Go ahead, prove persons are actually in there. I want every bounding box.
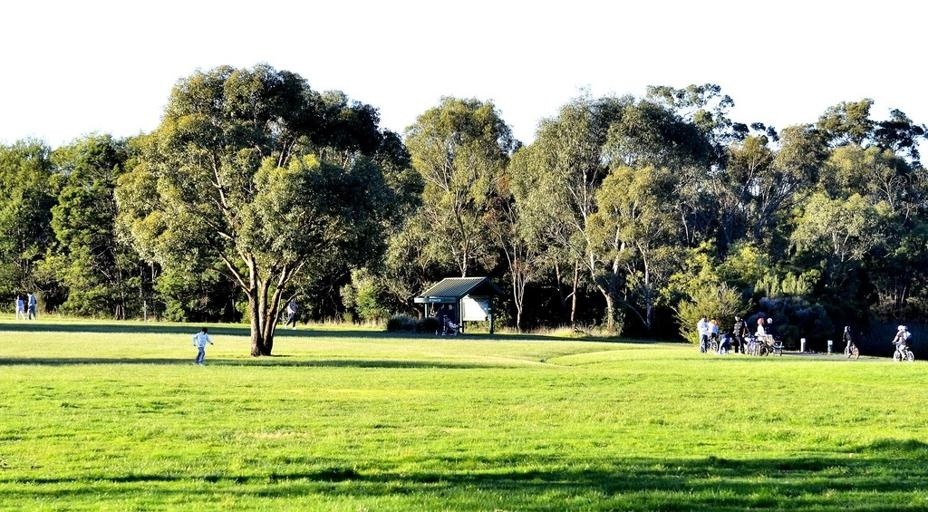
[843,325,856,358]
[892,325,911,360]
[192,327,214,368]
[26,291,37,320]
[284,297,298,330]
[15,293,28,321]
[695,313,784,355]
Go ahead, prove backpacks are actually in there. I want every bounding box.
[903,331,912,341]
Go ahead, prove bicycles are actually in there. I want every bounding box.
[891,341,914,362]
[706,331,784,360]
[841,340,860,361]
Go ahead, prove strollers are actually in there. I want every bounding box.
[442,314,463,338]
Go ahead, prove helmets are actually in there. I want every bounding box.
[897,325,908,330]
